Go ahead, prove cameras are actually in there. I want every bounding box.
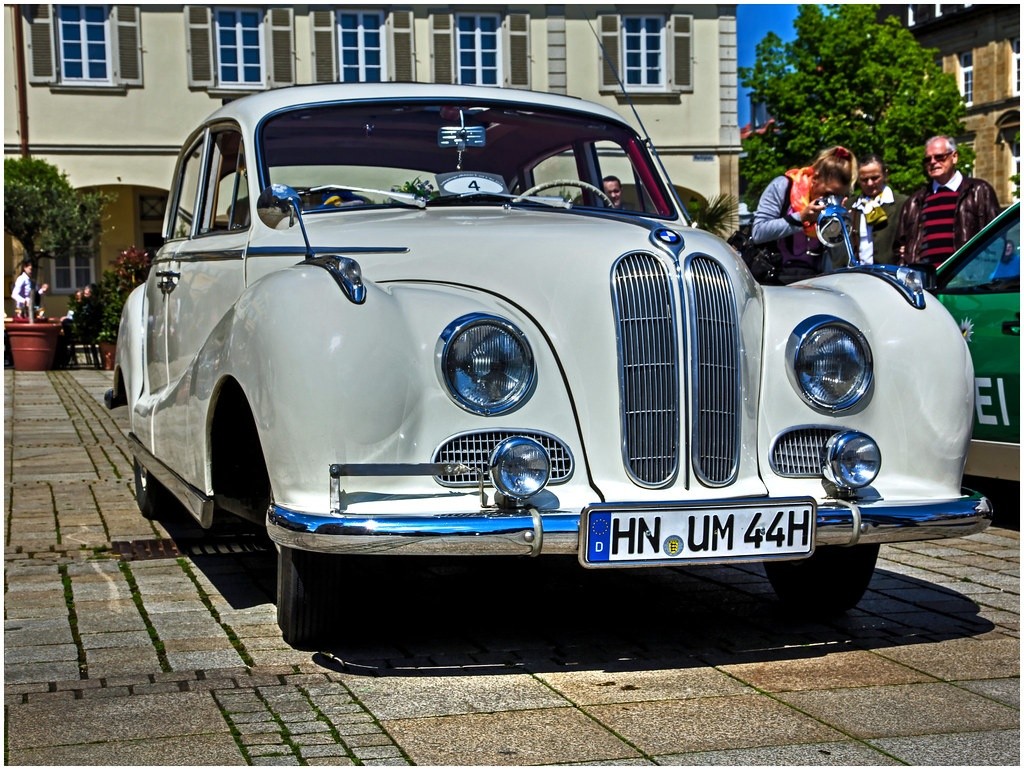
[817,194,842,209]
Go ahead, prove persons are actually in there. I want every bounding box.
[752,136,1020,287]
[603,175,634,210]
[10,260,109,369]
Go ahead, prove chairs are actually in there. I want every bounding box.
[67,342,101,370]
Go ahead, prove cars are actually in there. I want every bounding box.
[930,198,1020,480]
[102,79,994,652]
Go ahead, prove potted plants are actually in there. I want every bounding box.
[4,156,118,371]
[98,268,132,370]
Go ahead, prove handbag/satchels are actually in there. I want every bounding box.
[727,175,793,285]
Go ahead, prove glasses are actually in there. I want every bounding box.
[923,151,952,165]
